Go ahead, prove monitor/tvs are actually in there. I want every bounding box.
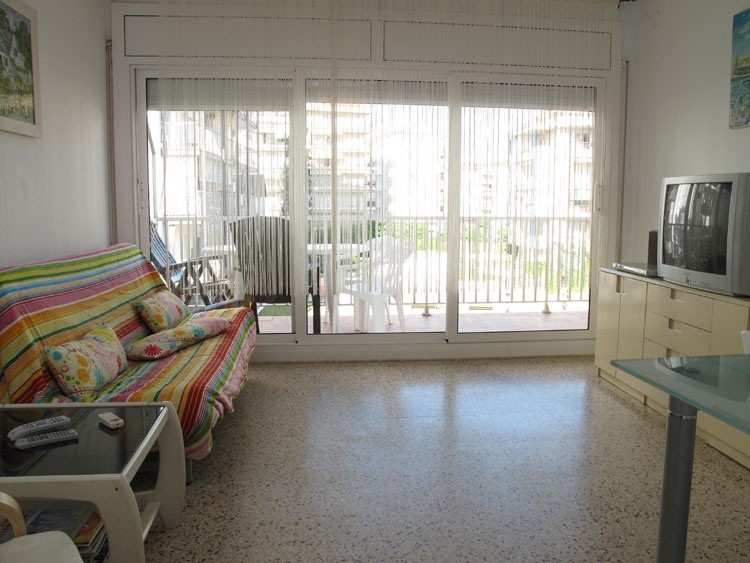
[658,171,750,297]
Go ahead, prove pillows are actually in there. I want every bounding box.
[125,316,231,360]
[43,322,128,403]
[132,290,193,333]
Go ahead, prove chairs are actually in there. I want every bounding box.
[327,236,417,331]
[149,220,234,306]
[229,217,322,335]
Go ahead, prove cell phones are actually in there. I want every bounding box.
[97,412,125,430]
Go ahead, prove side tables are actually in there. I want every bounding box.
[0,403,188,563]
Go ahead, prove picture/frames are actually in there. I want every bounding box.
[0,0,43,139]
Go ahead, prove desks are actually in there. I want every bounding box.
[202,243,370,327]
[610,355,750,563]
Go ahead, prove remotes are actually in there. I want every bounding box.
[14,429,78,449]
[6,415,71,442]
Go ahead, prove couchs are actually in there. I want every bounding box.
[0,243,257,484]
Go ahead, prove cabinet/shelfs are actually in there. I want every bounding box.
[594,271,647,406]
[644,283,715,439]
[713,299,750,469]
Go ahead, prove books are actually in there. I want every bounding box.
[0,509,109,563]
